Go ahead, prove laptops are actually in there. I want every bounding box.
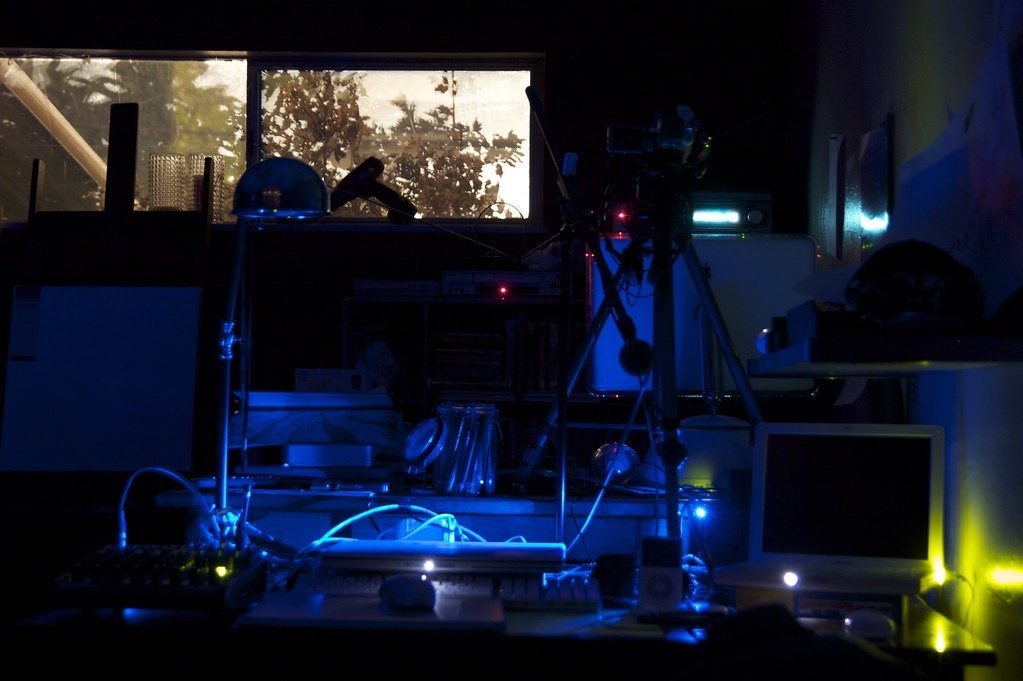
[586,231,817,398]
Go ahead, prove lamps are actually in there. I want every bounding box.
[208,156,326,564]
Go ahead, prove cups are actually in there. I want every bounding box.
[188,154,225,225]
[149,151,185,212]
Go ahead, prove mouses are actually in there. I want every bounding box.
[842,610,896,638]
[377,573,437,614]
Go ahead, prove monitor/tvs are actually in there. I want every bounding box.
[749,422,948,592]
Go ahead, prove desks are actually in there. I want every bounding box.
[0,557,998,681]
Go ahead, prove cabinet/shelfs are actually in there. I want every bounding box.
[582,234,1023,607]
[335,293,583,394]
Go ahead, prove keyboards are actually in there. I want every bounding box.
[318,566,601,615]
